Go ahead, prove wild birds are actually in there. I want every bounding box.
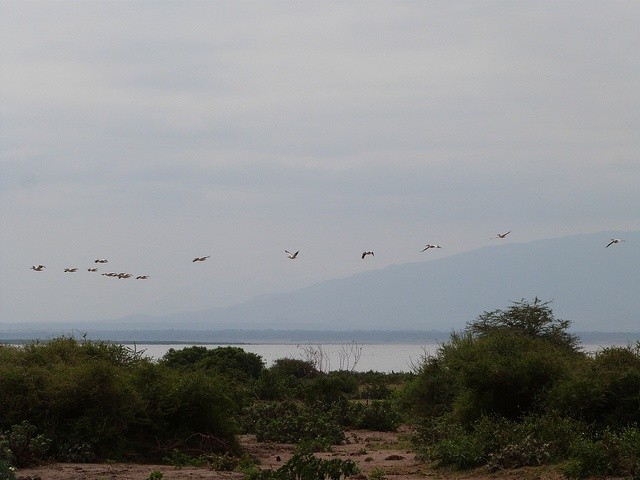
[420,244,441,252]
[95,259,108,263]
[361,251,375,258]
[284,250,300,259]
[118,273,132,279]
[101,272,115,276]
[605,239,626,248]
[88,268,98,272]
[488,231,511,239]
[64,268,77,272]
[30,265,46,271]
[114,272,125,277]
[136,275,149,279]
[192,256,210,262]
